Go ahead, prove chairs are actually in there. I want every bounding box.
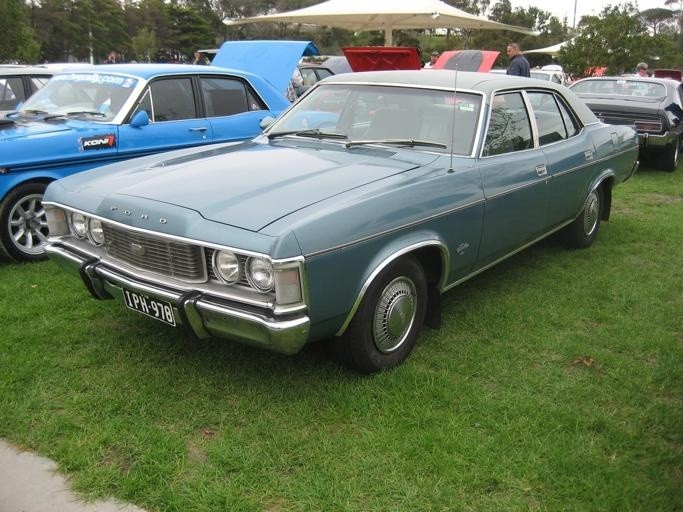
[363,95,514,159]
[205,89,256,119]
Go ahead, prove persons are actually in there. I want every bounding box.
[286,68,302,105]
[189,44,211,66]
[421,50,441,69]
[635,61,654,95]
[504,43,530,78]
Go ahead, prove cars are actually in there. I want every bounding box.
[0,46,569,122]
[41,70,640,373]
[0,44,339,263]
[536,68,682,172]
